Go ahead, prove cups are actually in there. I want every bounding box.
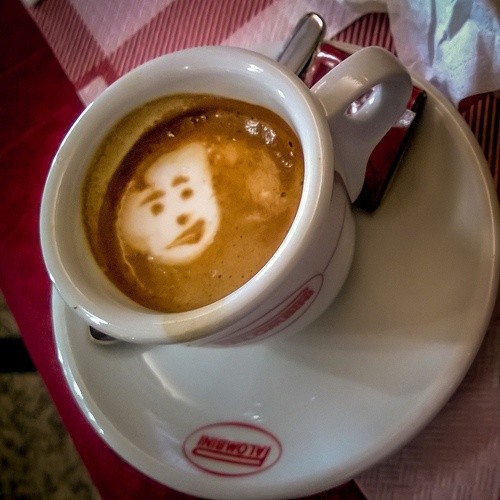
[39,44,414,352]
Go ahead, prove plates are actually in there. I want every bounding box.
[50,39,500,500]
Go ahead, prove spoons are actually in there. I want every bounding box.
[88,11,327,346]
